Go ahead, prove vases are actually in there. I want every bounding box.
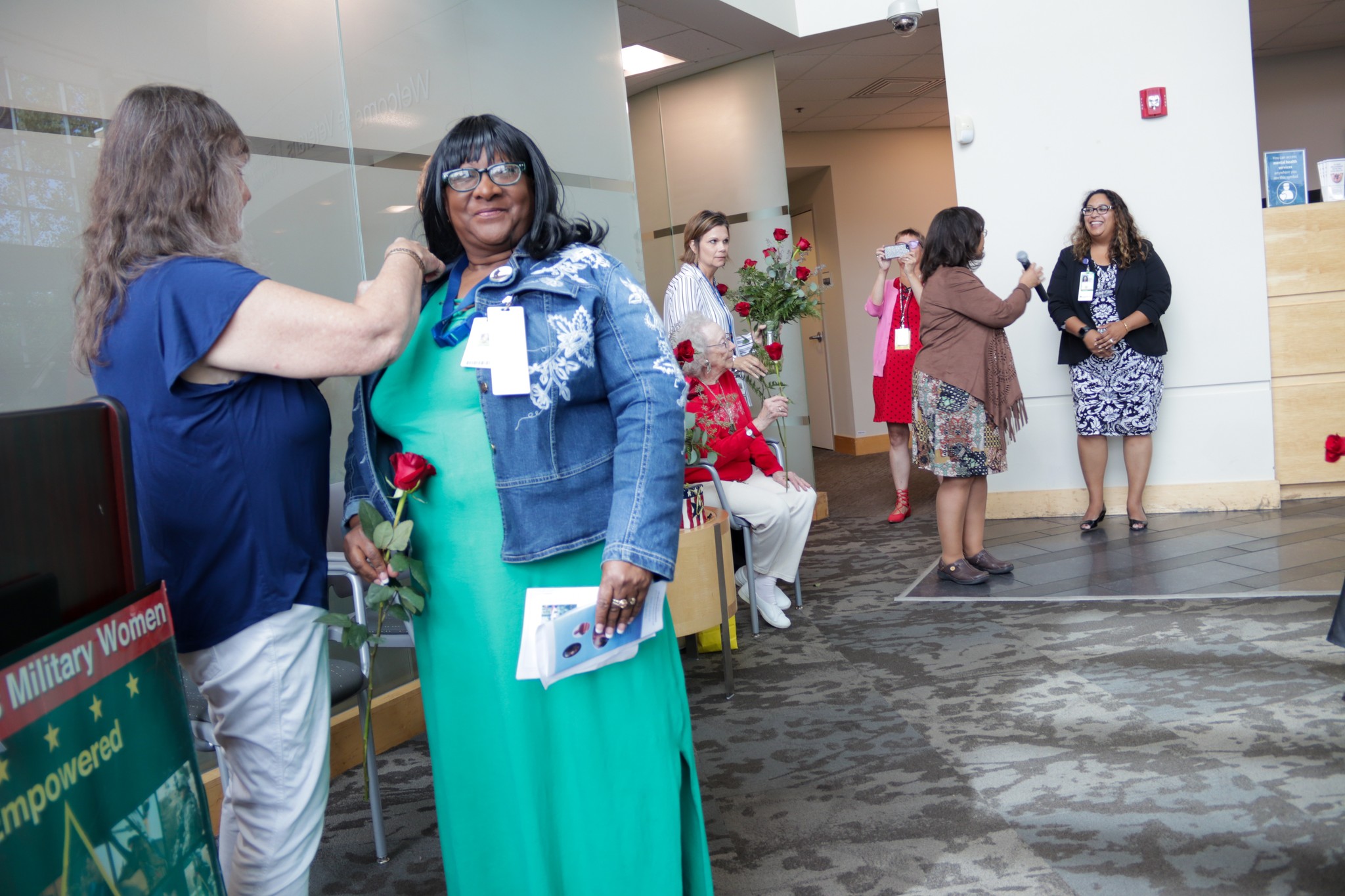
[760,325,782,376]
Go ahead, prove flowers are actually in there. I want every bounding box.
[314,452,435,802]
[674,339,726,488]
[1325,434,1345,463]
[733,300,786,453]
[764,341,791,493]
[714,230,823,320]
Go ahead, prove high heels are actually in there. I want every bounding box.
[1126,505,1148,530]
[1080,504,1106,530]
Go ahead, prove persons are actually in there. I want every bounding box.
[913,206,1046,584]
[864,228,946,523]
[1047,189,1172,532]
[79,85,448,896]
[1082,276,1088,281]
[342,111,719,896]
[664,210,819,630]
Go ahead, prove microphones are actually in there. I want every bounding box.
[1016,250,1049,303]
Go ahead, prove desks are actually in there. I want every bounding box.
[667,507,738,701]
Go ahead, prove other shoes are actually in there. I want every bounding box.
[937,557,989,584]
[734,565,791,610]
[888,489,911,523]
[738,584,791,629]
[969,549,1015,572]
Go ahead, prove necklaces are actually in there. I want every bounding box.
[698,376,737,435]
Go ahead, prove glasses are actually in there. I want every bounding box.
[704,332,732,349]
[896,240,923,249]
[438,162,527,192]
[1081,205,1116,216]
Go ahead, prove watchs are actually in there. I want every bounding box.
[744,426,756,439]
[1078,325,1094,340]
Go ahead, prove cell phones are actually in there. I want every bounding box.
[884,244,909,260]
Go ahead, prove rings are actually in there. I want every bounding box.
[612,597,627,609]
[779,407,782,411]
[750,365,753,369]
[1109,340,1114,343]
[627,598,636,605]
[875,255,879,257]
[907,258,909,261]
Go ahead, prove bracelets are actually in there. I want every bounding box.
[387,249,426,272]
[1120,320,1128,334]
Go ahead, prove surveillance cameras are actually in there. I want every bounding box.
[886,0,923,38]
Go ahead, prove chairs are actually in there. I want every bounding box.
[327,479,416,650]
[681,440,802,637]
[182,559,387,869]
